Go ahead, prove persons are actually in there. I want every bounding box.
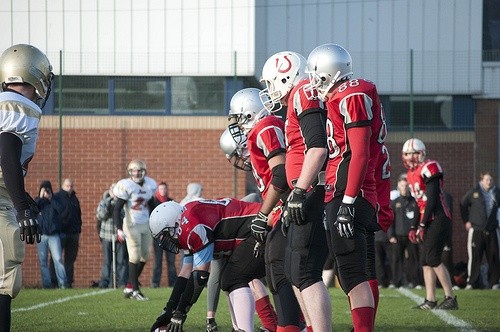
[258,50,333,332]
[220,126,278,332]
[96,183,204,288]
[229,88,300,332]
[0,44,54,332]
[149,198,264,332]
[303,43,386,332]
[113,160,158,301]
[49,178,82,288]
[402,138,458,311]
[34,180,66,289]
[367,140,453,325]
[459,173,500,289]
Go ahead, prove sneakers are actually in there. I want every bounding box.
[130,292,145,301]
[416,299,438,311]
[123,291,144,298]
[435,295,459,310]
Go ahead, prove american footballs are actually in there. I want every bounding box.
[152,326,171,332]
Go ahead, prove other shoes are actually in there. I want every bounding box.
[258,327,270,332]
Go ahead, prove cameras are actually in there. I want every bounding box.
[44,188,52,194]
[110,201,117,206]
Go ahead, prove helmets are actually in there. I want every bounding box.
[127,159,146,182]
[0,44,54,109]
[402,138,426,170]
[220,126,252,170]
[149,201,183,254]
[302,44,353,102]
[259,51,307,113]
[228,88,271,144]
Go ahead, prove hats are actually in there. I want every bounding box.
[41,180,51,189]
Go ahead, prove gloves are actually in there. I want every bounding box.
[247,211,268,242]
[282,187,306,237]
[150,312,173,332]
[254,241,265,258]
[106,197,113,208]
[334,202,355,238]
[205,318,218,332]
[14,203,43,244]
[408,229,419,244]
[416,225,426,244]
[165,310,187,332]
